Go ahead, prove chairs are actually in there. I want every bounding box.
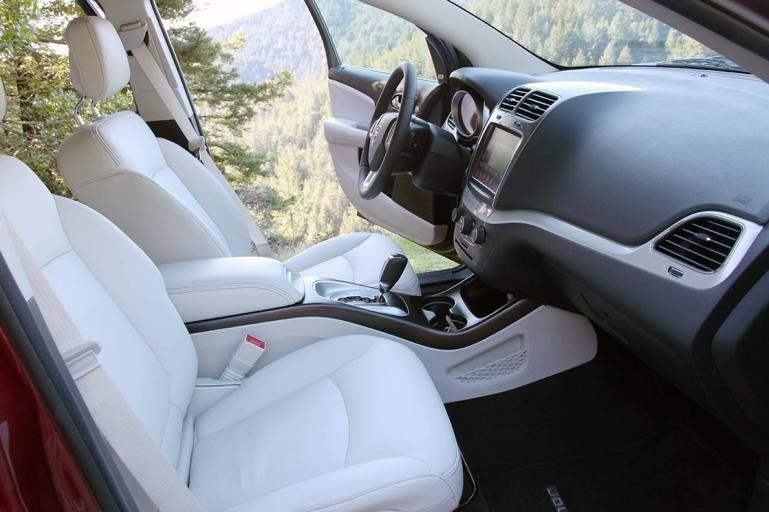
[0,79,464,511]
[56,15,421,321]
